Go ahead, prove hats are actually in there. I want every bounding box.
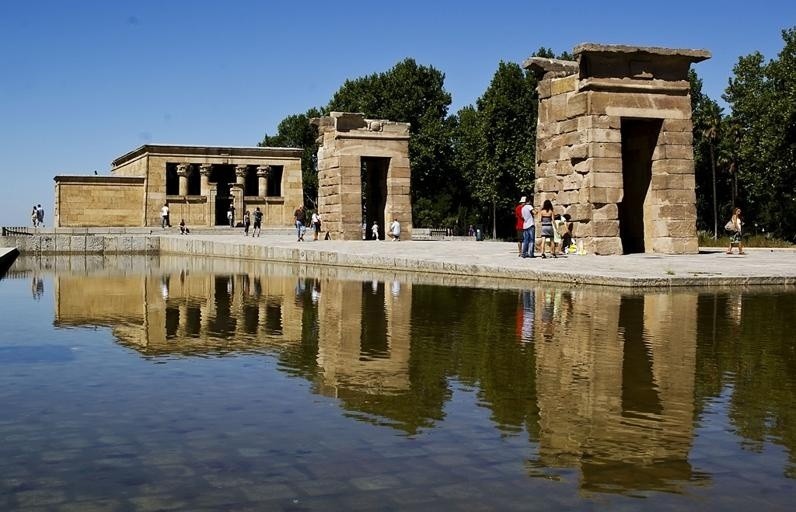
[519,196,527,203]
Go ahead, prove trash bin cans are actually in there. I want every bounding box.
[476,229,481,241]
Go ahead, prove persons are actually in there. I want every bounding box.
[362,222,367,231]
[726,290,742,343]
[310,210,322,241]
[251,207,263,237]
[31,275,44,302]
[225,275,262,305]
[468,224,474,236]
[386,218,401,241]
[243,210,250,236]
[371,280,379,295]
[726,207,747,255]
[515,287,574,356]
[227,204,236,225]
[37,204,45,227]
[160,272,170,305]
[160,203,172,228]
[515,196,573,258]
[30,206,39,228]
[391,280,401,302]
[293,278,320,310]
[324,230,332,241]
[178,218,187,235]
[371,221,380,241]
[293,205,306,242]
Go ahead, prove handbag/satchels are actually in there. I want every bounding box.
[724,221,738,232]
[314,219,319,225]
[32,213,37,221]
